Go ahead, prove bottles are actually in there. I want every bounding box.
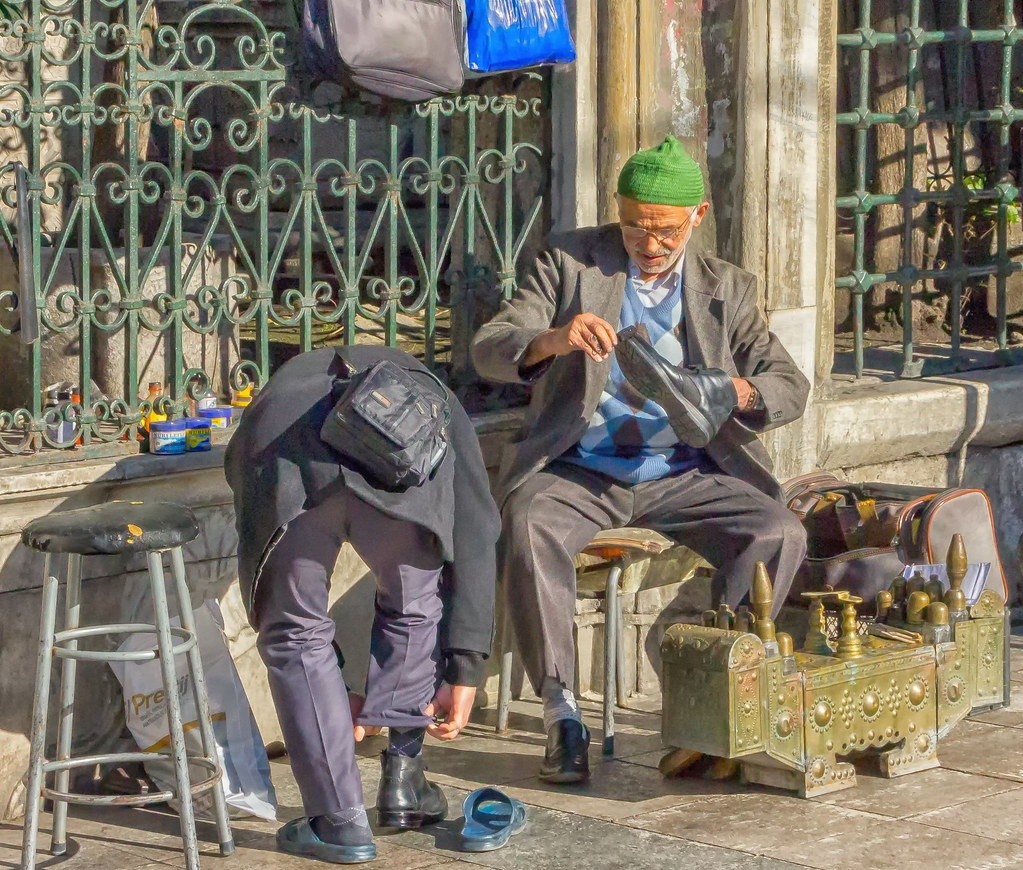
[43,388,82,444]
[141,382,168,433]
[195,376,217,409]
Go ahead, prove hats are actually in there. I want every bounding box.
[616,132,704,207]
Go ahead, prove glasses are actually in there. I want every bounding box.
[619,206,698,243]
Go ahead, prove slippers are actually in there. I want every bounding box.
[276,816,377,863]
[458,786,528,851]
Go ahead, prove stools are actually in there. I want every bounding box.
[497,525,686,755]
[20,501,234,870]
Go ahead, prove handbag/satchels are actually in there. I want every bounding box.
[319,359,452,494]
[456,0,577,79]
[780,471,1008,616]
[299,0,464,103]
[108,598,278,824]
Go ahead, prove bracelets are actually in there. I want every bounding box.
[739,388,756,414]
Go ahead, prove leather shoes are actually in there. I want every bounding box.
[537,719,591,783]
[614,325,738,449]
[375,747,448,829]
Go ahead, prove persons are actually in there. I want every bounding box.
[224,345,501,863]
[469,137,809,782]
